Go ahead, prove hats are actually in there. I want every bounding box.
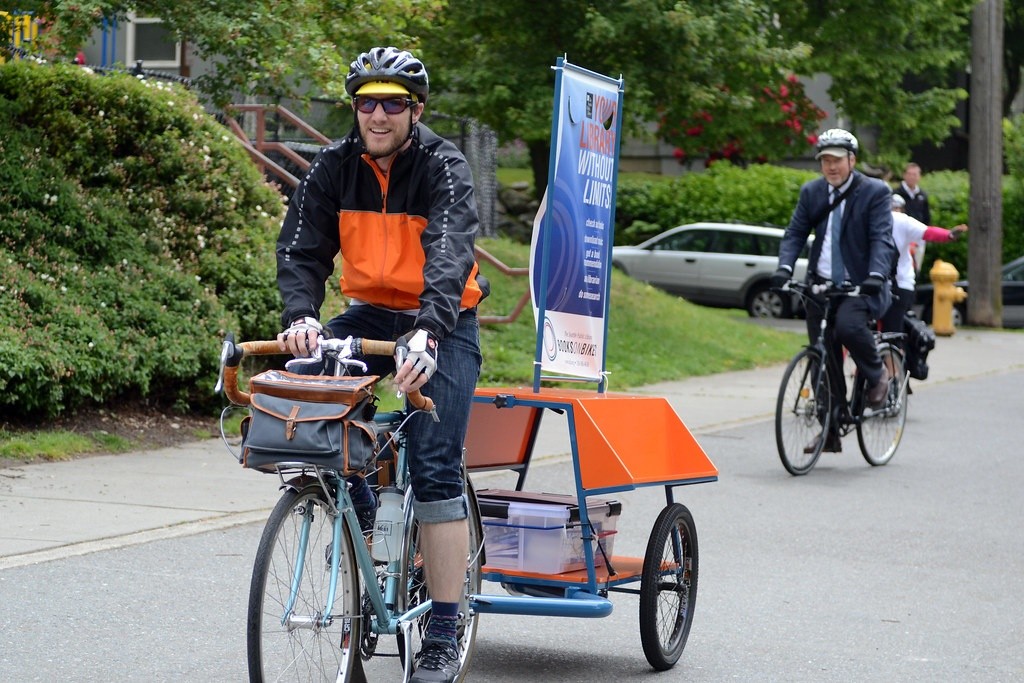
[815,146,854,160]
[355,63,418,103]
[891,193,906,208]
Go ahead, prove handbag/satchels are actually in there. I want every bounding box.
[903,312,935,379]
[240,366,380,473]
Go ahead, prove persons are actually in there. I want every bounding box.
[894,162,931,225]
[882,210,967,417]
[770,128,900,454]
[890,192,904,212]
[276,45,491,683]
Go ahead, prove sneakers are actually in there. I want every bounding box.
[410,634,461,683]
[325,493,379,568]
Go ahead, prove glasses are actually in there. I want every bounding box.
[354,96,418,115]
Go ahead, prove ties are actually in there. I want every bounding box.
[832,189,845,286]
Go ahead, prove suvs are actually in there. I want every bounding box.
[612,222,816,320]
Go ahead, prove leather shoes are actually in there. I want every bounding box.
[868,366,889,406]
[803,431,841,453]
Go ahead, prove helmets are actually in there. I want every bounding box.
[816,129,858,152]
[345,47,429,105]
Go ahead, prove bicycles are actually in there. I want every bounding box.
[771,285,912,476]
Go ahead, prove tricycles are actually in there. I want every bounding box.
[212,333,719,683]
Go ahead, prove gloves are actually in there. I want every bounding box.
[858,275,884,296]
[770,268,791,292]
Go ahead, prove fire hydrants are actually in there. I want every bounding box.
[928,260,969,339]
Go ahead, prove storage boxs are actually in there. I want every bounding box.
[478,488,622,575]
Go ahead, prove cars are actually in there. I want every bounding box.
[916,256,1023,329]
